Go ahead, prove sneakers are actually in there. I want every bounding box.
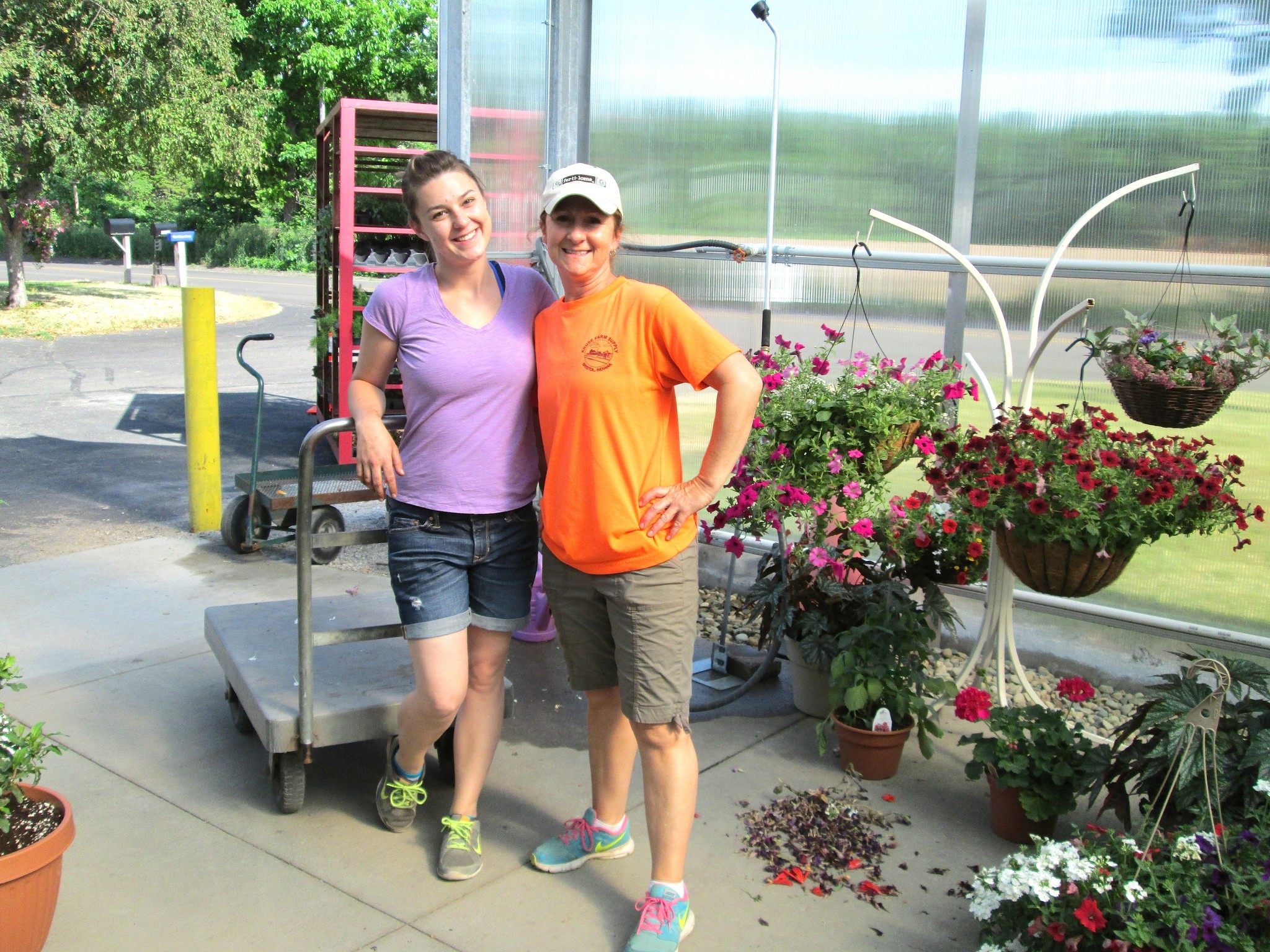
[436,813,484,880]
[375,734,426,833]
[528,807,634,874]
[625,880,695,952]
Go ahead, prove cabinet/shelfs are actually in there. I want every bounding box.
[314,97,439,466]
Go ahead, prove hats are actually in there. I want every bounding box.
[539,163,623,223]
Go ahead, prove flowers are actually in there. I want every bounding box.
[699,281,1269,952]
[0,650,72,836]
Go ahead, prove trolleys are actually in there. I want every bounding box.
[201,415,514,814]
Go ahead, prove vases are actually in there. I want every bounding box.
[0,781,76,952]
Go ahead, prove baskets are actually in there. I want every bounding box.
[1099,247,1249,428]
[994,383,1141,597]
[804,286,921,476]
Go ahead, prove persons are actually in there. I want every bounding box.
[530,163,764,952]
[347,150,563,882]
